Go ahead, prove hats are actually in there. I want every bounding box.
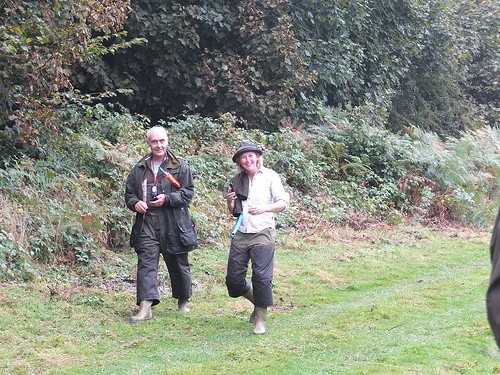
[232,142,263,163]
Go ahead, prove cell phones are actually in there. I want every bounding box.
[229,183,233,192]
[151,196,157,201]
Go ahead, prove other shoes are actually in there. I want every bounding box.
[177,299,190,313]
[129,301,152,323]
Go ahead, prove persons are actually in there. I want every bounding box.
[125,126,197,322]
[225,141,291,334]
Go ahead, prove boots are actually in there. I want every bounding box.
[253,306,268,334]
[246,283,261,323]
